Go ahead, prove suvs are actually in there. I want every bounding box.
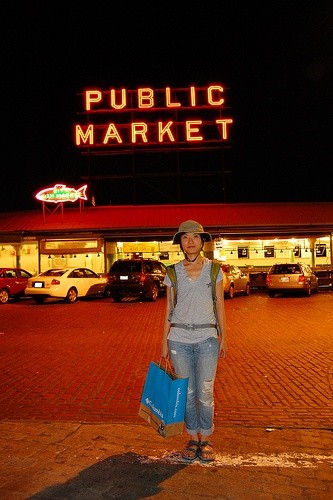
[106,257,167,302]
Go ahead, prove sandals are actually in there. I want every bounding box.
[182,440,200,460]
[199,439,216,461]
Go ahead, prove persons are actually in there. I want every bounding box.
[161,220,226,461]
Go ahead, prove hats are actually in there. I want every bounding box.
[172,220,212,245]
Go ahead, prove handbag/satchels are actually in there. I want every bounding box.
[138,355,188,439]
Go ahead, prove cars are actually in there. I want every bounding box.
[219,262,252,298]
[24,267,110,304]
[0,268,35,303]
[266,262,319,296]
